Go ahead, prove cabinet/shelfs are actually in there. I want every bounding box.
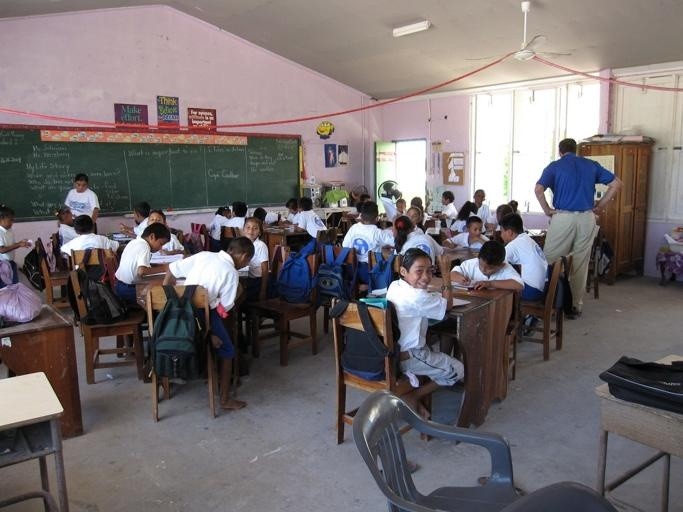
[576,141,652,287]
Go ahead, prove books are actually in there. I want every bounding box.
[592,134,652,143]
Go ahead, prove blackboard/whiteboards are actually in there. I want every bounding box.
[0,123,303,224]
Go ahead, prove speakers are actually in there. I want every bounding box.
[304,185,321,209]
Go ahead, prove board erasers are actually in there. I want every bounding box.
[166,208,180,210]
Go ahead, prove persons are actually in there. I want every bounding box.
[535,139,624,317]
[328,146,334,166]
[339,147,346,164]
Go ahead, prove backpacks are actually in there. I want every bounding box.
[545,253,574,316]
[277,236,317,303]
[22,239,49,292]
[81,248,116,293]
[42,235,60,274]
[187,222,210,256]
[368,251,396,301]
[596,235,615,277]
[66,269,128,325]
[317,245,348,307]
[148,283,207,384]
[330,297,401,383]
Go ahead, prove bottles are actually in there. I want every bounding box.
[378,213,383,220]
[275,211,287,222]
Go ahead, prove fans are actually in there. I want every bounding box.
[462,1,574,65]
[377,180,403,206]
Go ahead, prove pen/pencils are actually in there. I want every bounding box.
[461,267,465,276]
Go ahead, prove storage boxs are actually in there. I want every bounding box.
[668,239,683,253]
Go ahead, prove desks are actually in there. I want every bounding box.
[429,276,515,404]
[0,372,68,512]
[1,305,85,441]
[657,252,683,286]
[430,290,494,444]
[595,352,682,510]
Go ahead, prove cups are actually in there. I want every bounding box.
[289,225,294,232]
[434,221,440,230]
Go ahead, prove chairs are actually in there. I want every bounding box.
[36,241,71,307]
[329,296,440,446]
[67,208,603,385]
[351,390,521,512]
[147,284,229,421]
[498,481,615,511]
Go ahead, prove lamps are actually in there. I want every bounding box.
[392,18,431,40]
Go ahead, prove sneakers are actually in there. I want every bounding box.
[568,308,584,320]
